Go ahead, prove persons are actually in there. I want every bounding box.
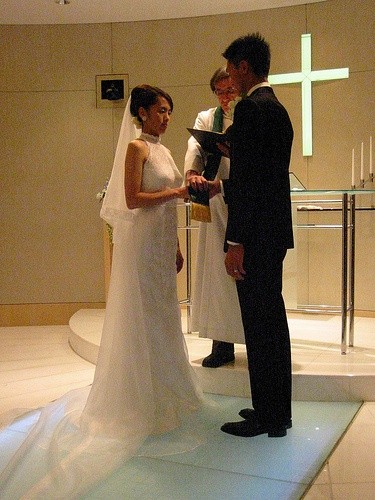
[183,67,245,369]
[184,32,293,438]
[0,83,224,468]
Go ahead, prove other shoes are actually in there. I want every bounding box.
[202,341,236,368]
[239,408,292,431]
[220,408,288,438]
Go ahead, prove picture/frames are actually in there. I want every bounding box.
[95,73,130,110]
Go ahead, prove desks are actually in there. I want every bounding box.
[161,186,374,355]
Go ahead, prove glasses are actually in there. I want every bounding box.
[212,89,236,98]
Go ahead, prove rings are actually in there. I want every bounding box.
[234,270,238,272]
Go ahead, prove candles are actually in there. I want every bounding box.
[369,136,373,177]
[352,148,355,187]
[360,142,364,181]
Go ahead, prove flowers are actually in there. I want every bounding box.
[224,93,243,121]
[96,175,111,204]
